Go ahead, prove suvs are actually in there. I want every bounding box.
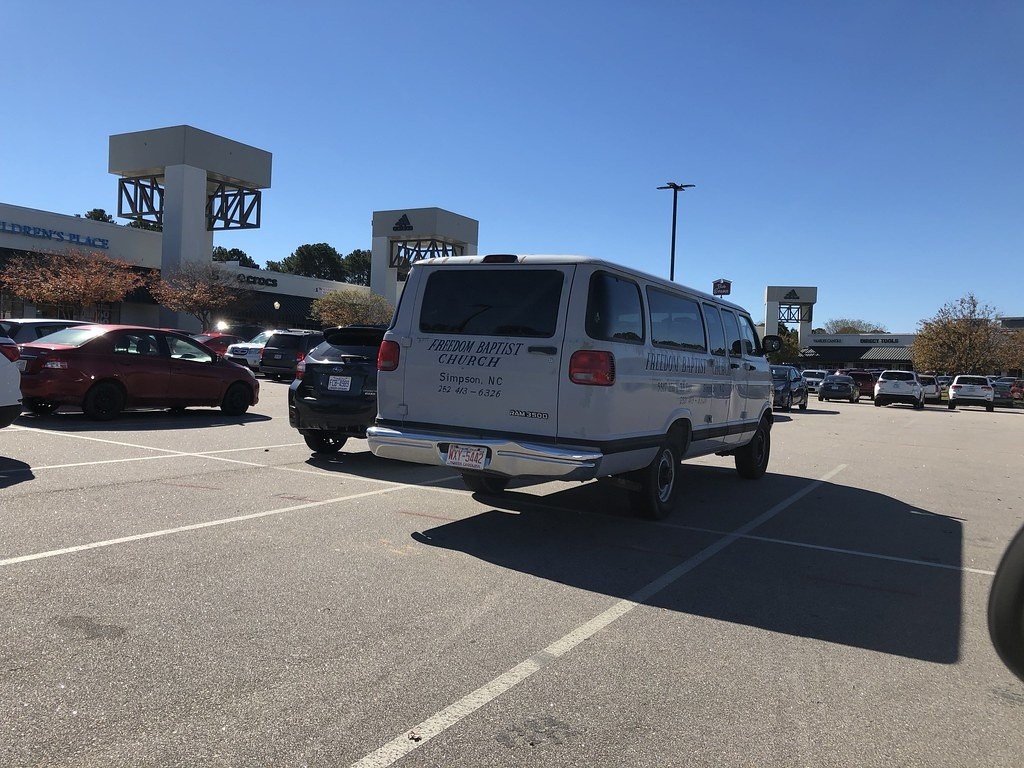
[287,326,389,454]
[223,329,284,373]
[916,374,942,404]
[0,323,23,429]
[874,370,927,410]
[258,329,325,381]
[846,372,878,400]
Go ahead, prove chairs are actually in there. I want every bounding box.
[116,338,130,352]
[136,341,150,354]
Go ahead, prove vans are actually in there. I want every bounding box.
[800,370,830,394]
[994,377,1021,390]
[365,253,783,519]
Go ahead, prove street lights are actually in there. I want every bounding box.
[656,181,697,284]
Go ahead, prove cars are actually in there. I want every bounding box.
[985,375,999,388]
[826,367,885,384]
[177,333,251,361]
[1011,380,1024,401]
[16,325,259,417]
[818,375,863,403]
[946,375,995,412]
[769,365,810,411]
[993,385,1014,409]
[937,375,954,390]
[0,318,104,347]
